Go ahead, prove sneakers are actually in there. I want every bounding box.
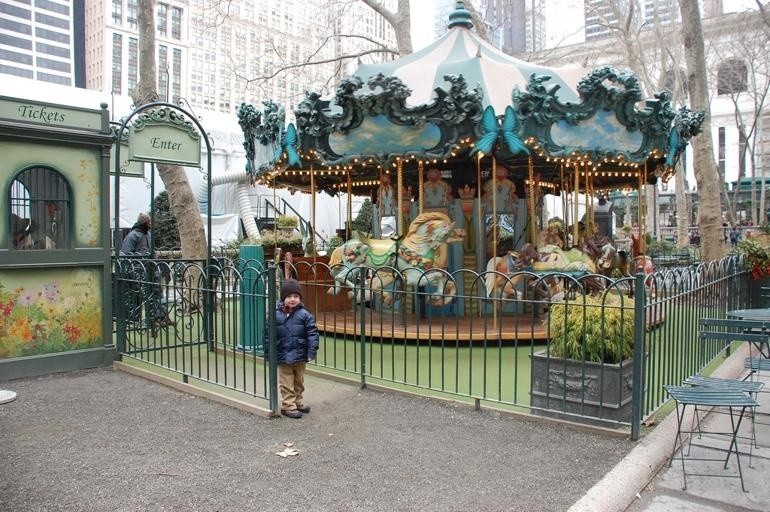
[298,406,311,413]
[281,410,303,417]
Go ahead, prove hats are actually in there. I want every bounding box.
[280,279,302,301]
[137,213,150,224]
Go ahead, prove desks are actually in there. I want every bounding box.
[725,309,770,323]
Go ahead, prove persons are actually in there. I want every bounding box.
[121,213,176,325]
[261,278,320,419]
[12,213,30,247]
[730,227,740,247]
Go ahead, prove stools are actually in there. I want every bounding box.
[661,359,770,493]
